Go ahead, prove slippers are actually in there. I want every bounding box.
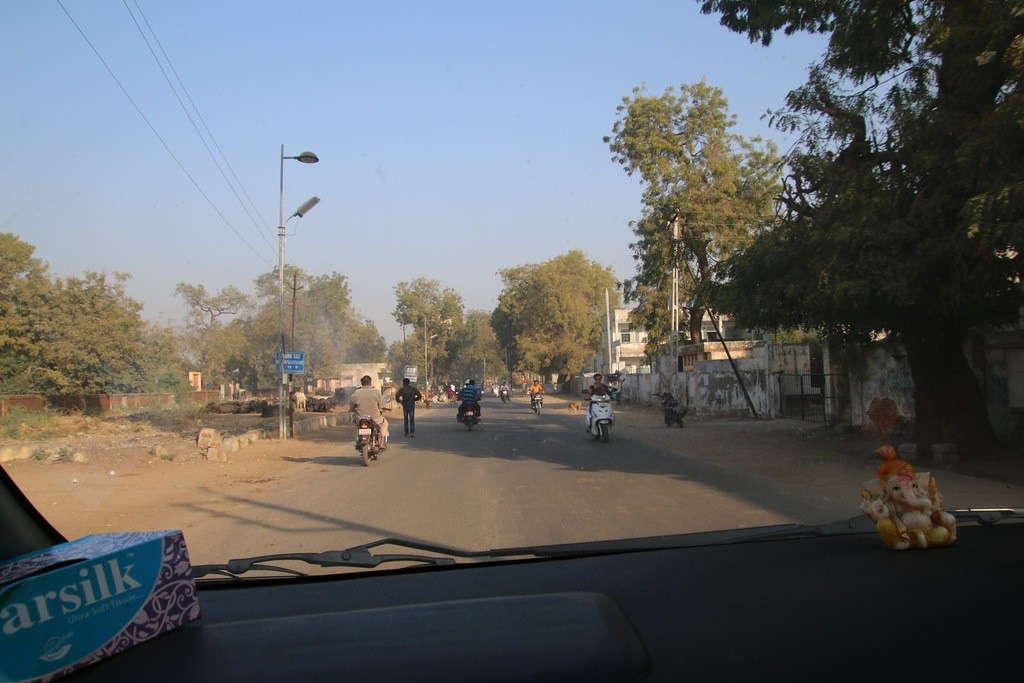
[355,439,361,446]
[379,444,388,450]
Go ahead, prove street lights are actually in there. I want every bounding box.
[424,314,436,407]
[278,143,319,442]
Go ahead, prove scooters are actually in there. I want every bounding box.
[581,386,618,443]
[491,384,502,399]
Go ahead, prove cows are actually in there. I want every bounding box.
[206,390,335,414]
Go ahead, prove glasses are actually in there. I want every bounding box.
[595,378,602,380]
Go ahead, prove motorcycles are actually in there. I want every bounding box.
[499,389,510,404]
[455,390,484,433]
[653,392,688,428]
[351,395,393,467]
[528,388,543,416]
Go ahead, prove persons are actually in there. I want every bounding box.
[446,382,456,400]
[588,374,614,426]
[529,380,545,409]
[498,382,511,401]
[349,375,388,450]
[396,378,422,437]
[457,380,482,422]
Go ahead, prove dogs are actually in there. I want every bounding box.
[568,400,584,414]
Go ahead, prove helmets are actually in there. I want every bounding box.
[465,379,470,384]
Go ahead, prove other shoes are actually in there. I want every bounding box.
[404,432,409,437]
[587,427,591,432]
[411,432,415,438]
